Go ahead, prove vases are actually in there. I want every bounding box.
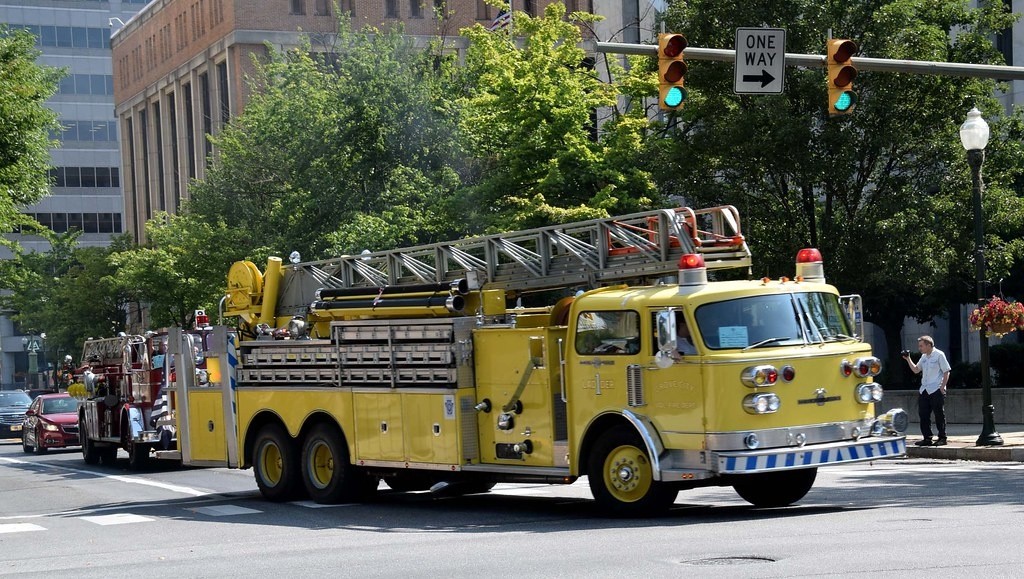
[988,320,1014,332]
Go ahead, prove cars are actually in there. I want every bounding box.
[0,389,34,441]
[22,392,85,455]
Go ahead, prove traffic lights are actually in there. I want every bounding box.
[657,33,688,112]
[826,38,858,116]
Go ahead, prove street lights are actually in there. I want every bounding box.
[959,108,1005,445]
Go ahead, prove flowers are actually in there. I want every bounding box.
[967,295,1024,338]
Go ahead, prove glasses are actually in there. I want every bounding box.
[163,344,167,346]
[918,338,924,341]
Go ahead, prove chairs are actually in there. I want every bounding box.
[44,400,54,410]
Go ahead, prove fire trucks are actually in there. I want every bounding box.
[161,203,909,519]
[66,309,240,469]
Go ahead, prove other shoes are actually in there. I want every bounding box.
[933,436,947,446]
[915,438,933,446]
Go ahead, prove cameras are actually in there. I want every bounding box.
[901,348,910,357]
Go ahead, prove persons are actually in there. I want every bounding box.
[903,335,951,446]
[655,311,698,359]
